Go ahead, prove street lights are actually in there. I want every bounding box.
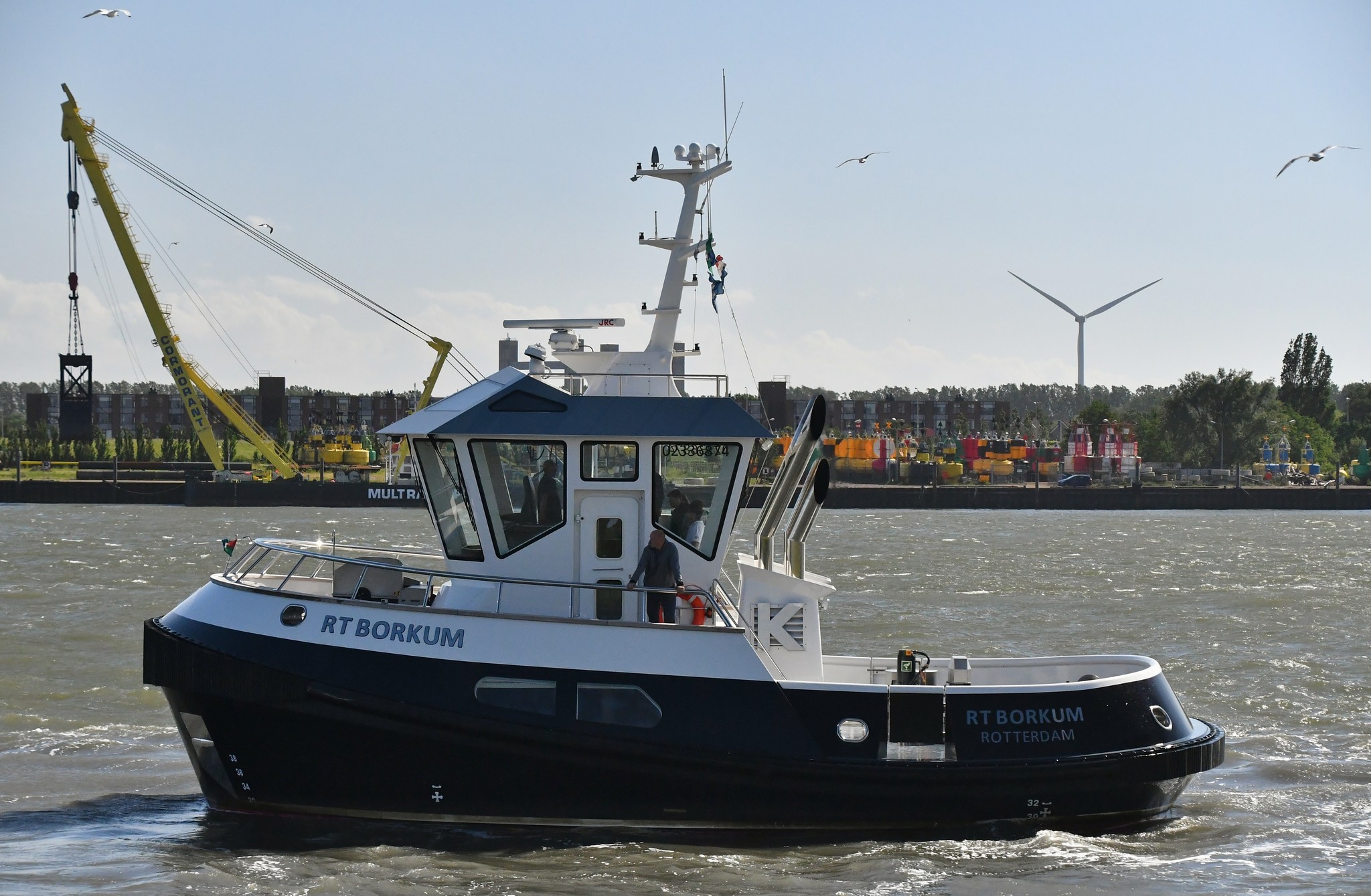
[394,398,398,422]
[914,388,919,439]
[1034,401,1039,440]
[1210,421,1223,469]
[745,387,748,412]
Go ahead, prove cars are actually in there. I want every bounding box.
[1057,474,1092,488]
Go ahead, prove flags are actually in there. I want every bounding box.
[222,538,237,557]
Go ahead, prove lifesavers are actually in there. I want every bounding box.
[658,592,705,625]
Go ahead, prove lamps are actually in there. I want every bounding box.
[524,343,551,379]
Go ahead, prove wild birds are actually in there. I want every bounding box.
[257,222,275,234]
[834,151,892,169]
[78,8,132,19]
[1274,143,1362,179]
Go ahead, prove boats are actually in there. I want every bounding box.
[140,141,1232,835]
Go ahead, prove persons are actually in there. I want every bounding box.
[683,500,705,549]
[666,489,690,539]
[626,530,685,625]
[536,459,564,525]
